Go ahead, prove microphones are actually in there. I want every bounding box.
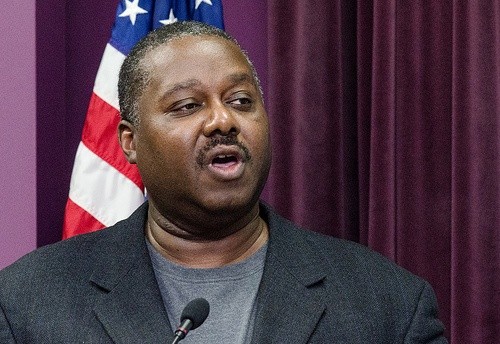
[169,299,211,344]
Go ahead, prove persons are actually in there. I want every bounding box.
[0,19,444,343]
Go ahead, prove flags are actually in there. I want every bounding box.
[63,0,226,238]
